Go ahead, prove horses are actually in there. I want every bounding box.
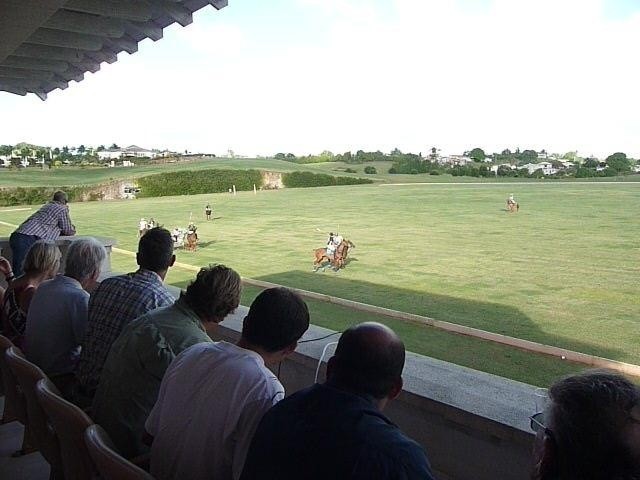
[507,199,519,212]
[313,237,355,272]
[138,228,198,252]
[206,206,211,220]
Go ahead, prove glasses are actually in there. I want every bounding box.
[529,412,553,437]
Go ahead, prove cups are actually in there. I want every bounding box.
[534,386,550,414]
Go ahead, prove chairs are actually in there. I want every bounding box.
[0,336,165,480]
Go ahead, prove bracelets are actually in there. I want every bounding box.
[5,271,13,275]
[5,276,14,282]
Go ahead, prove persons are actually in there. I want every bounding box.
[0,251,15,335]
[531,367,639,480]
[142,285,310,480]
[75,224,176,399]
[1,239,64,354]
[206,203,210,210]
[8,190,76,277]
[508,193,515,207]
[327,233,338,245]
[139,218,147,232]
[25,236,108,384]
[239,321,435,479]
[95,262,244,461]
